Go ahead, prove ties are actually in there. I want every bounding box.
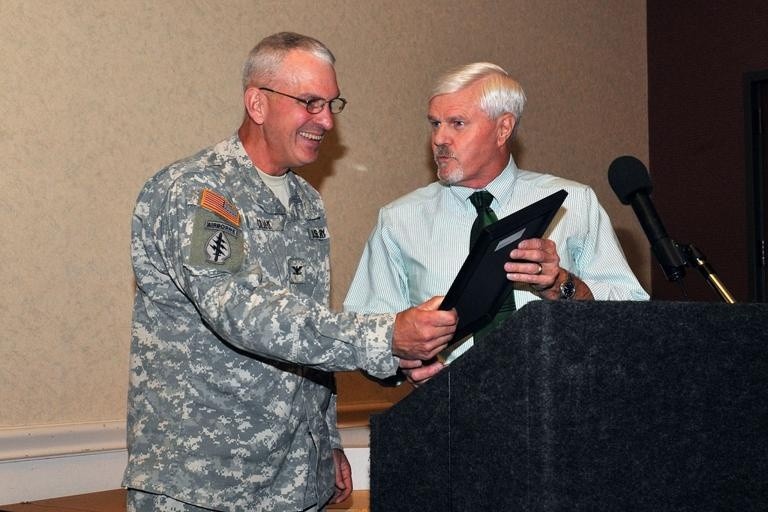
[469,192,515,344]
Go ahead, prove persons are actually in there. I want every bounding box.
[121,30,459,510]
[341,63,649,389]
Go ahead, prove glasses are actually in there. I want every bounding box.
[259,86,347,114]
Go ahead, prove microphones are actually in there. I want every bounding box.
[608,156,693,282]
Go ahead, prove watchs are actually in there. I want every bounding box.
[560,269,576,301]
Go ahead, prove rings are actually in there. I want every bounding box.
[538,263,543,275]
